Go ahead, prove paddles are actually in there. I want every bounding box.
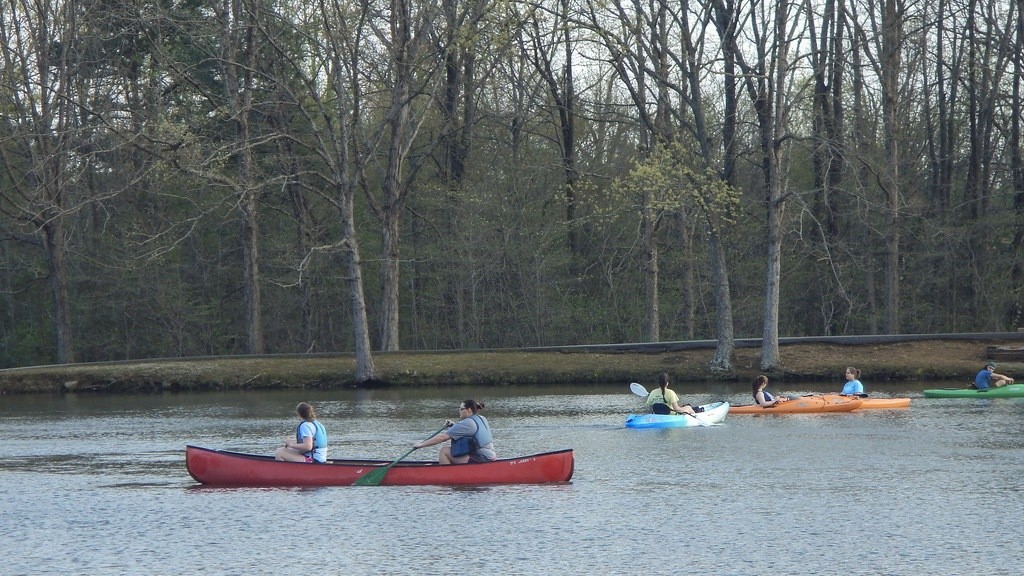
[284,441,288,449]
[352,422,451,487]
[804,394,868,398]
[629,382,712,427]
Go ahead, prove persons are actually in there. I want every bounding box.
[646,372,695,415]
[274,401,327,464]
[751,374,780,407]
[837,366,863,396]
[975,362,1014,388]
[413,399,496,464]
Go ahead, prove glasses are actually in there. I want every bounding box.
[459,407,471,411]
[988,366,995,371]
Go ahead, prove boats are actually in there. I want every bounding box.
[624,399,731,429]
[728,392,866,415]
[855,394,912,409]
[185,444,576,489]
[921,382,1024,399]
